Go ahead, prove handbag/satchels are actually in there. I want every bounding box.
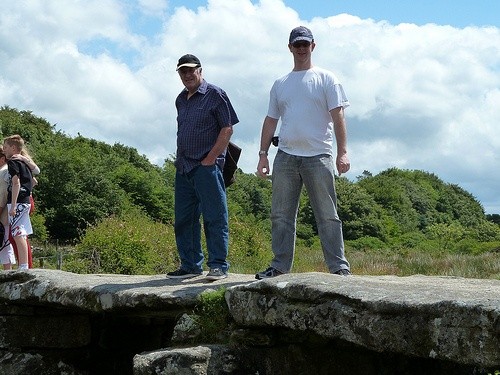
[222,140,242,188]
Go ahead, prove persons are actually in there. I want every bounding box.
[165,54,233,280]
[3,135,31,270]
[255,26,351,279]
[0,142,40,269]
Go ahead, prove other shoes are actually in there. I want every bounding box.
[11,268,29,274]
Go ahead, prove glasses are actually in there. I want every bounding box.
[290,40,312,48]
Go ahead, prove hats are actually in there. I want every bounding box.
[175,53,201,71]
[289,26,313,45]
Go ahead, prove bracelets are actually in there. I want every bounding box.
[258,150,268,155]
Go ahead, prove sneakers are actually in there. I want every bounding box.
[335,269,351,276]
[255,267,283,280]
[206,268,228,279]
[166,267,200,279]
